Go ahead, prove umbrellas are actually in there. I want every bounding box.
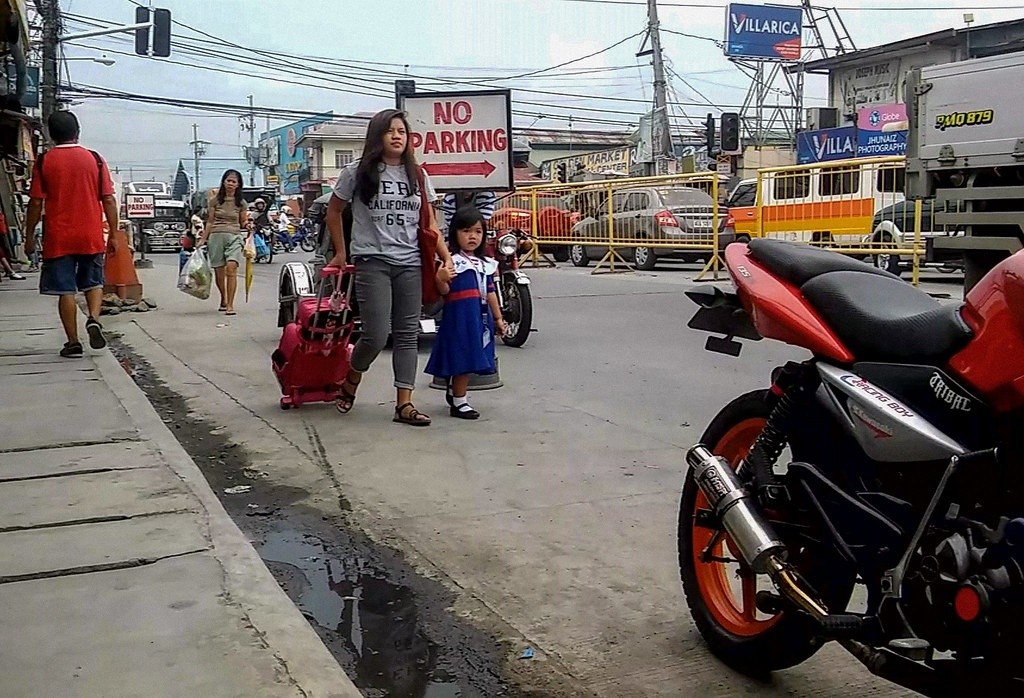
[245,231,252,303]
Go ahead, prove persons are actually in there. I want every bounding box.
[0,207,27,280]
[196,169,252,315]
[252,194,278,255]
[441,192,495,235]
[424,206,506,416]
[25,110,119,358]
[191,205,205,236]
[278,206,300,253]
[326,109,455,423]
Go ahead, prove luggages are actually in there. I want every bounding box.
[272,263,358,408]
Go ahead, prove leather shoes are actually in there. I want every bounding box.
[450,403,480,418]
[446,375,453,406]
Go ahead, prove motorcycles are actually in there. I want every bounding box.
[273,217,319,255]
[277,227,540,348]
[247,218,279,264]
[676,237,1024,698]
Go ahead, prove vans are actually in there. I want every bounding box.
[723,153,905,262]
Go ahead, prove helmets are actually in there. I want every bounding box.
[248,198,266,208]
[281,205,292,214]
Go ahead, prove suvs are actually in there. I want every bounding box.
[569,185,728,272]
[488,190,576,262]
[303,191,353,251]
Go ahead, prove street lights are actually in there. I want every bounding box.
[41,56,116,152]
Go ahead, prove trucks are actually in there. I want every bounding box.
[118,178,202,254]
[862,51,1024,277]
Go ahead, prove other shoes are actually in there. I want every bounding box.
[86,320,106,349]
[219,302,236,315]
[60,342,83,357]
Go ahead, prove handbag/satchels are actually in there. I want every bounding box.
[243,230,257,260]
[254,214,269,226]
[253,233,270,260]
[177,246,214,300]
[417,228,441,305]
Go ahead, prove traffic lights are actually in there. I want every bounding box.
[557,163,566,183]
[576,163,586,171]
[702,113,715,151]
[720,112,739,151]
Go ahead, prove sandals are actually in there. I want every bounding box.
[393,403,432,423]
[336,372,362,413]
